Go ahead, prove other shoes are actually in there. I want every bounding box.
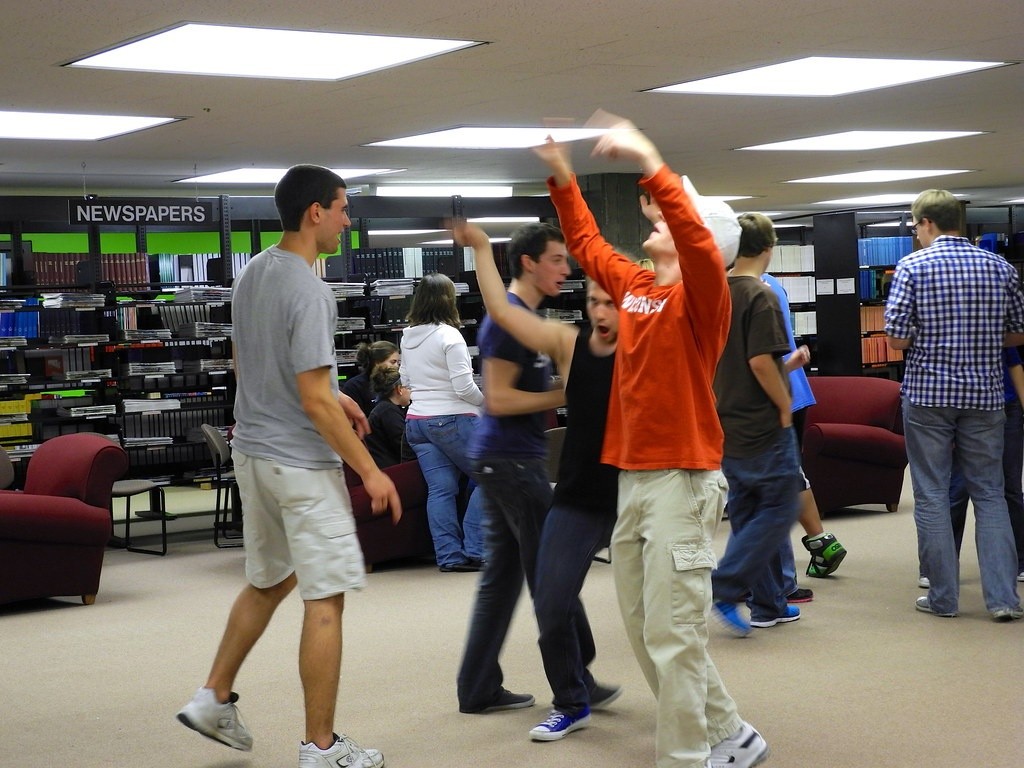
[440,560,482,572]
[1017,572,1024,581]
[918,575,930,588]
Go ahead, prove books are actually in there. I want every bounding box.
[310,243,512,388]
[0,250,251,462]
[858,237,924,367]
[767,245,817,335]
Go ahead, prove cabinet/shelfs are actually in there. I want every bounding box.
[1,194,1023,486]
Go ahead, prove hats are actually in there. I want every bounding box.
[680,174,742,268]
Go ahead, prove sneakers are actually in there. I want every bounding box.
[992,605,1023,618]
[299,731,384,768]
[915,596,933,612]
[530,703,592,740]
[176,686,252,752]
[711,598,750,637]
[482,686,534,711]
[751,604,802,627]
[801,531,847,577]
[784,588,813,603]
[587,684,624,710]
[709,720,771,768]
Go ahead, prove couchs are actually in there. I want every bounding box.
[801,375,908,518]
[344,429,432,573]
[0,432,128,608]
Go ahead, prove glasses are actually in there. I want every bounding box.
[910,218,932,235]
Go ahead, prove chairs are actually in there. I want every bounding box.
[0,445,15,489]
[83,432,167,555]
[201,423,245,548]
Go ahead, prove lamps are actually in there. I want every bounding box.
[368,183,516,198]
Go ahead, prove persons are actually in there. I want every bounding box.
[710,212,802,639]
[400,272,486,572]
[533,109,768,768]
[176,165,402,768]
[735,345,811,603]
[760,272,847,577]
[884,189,1024,623]
[341,340,399,418]
[364,364,418,469]
[457,223,620,714]
[446,218,621,741]
